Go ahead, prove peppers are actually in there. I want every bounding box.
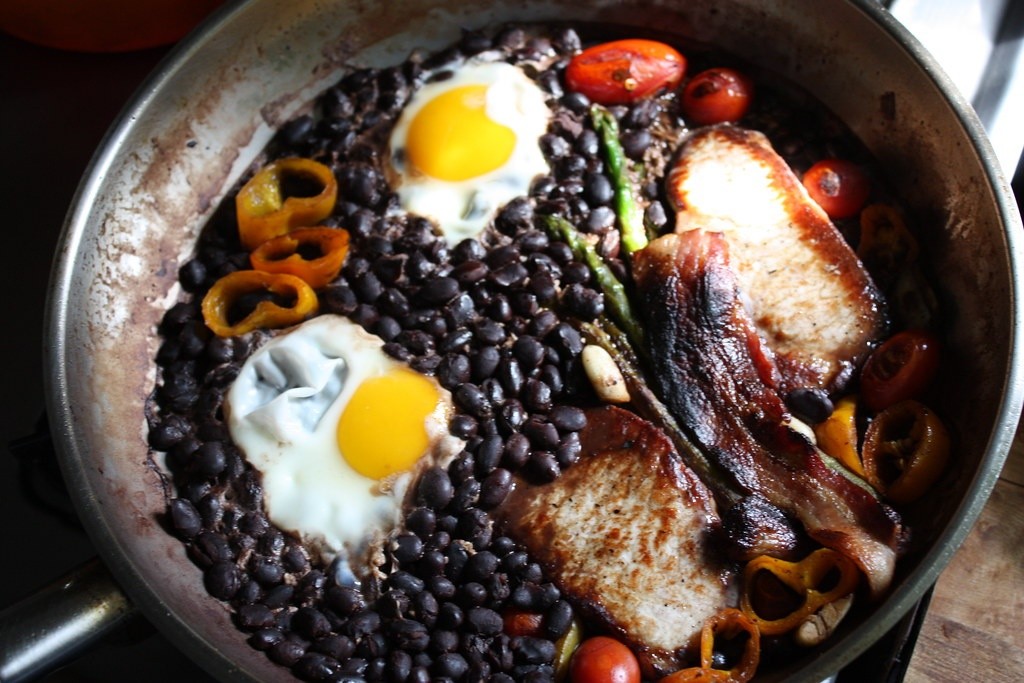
[236,158,337,241]
[253,229,349,285]
[862,408,945,502]
[861,335,935,402]
[201,271,318,336]
[741,547,855,631]
[698,610,760,682]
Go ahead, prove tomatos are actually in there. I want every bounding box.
[685,68,748,121]
[805,162,867,215]
[566,40,684,101]
[571,638,640,683]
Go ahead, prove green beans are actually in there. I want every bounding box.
[151,20,676,683]
[592,104,646,255]
[547,215,648,360]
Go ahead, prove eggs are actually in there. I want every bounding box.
[227,314,451,554]
[390,51,551,237]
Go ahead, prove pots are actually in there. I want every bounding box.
[0,0,1024,683]
